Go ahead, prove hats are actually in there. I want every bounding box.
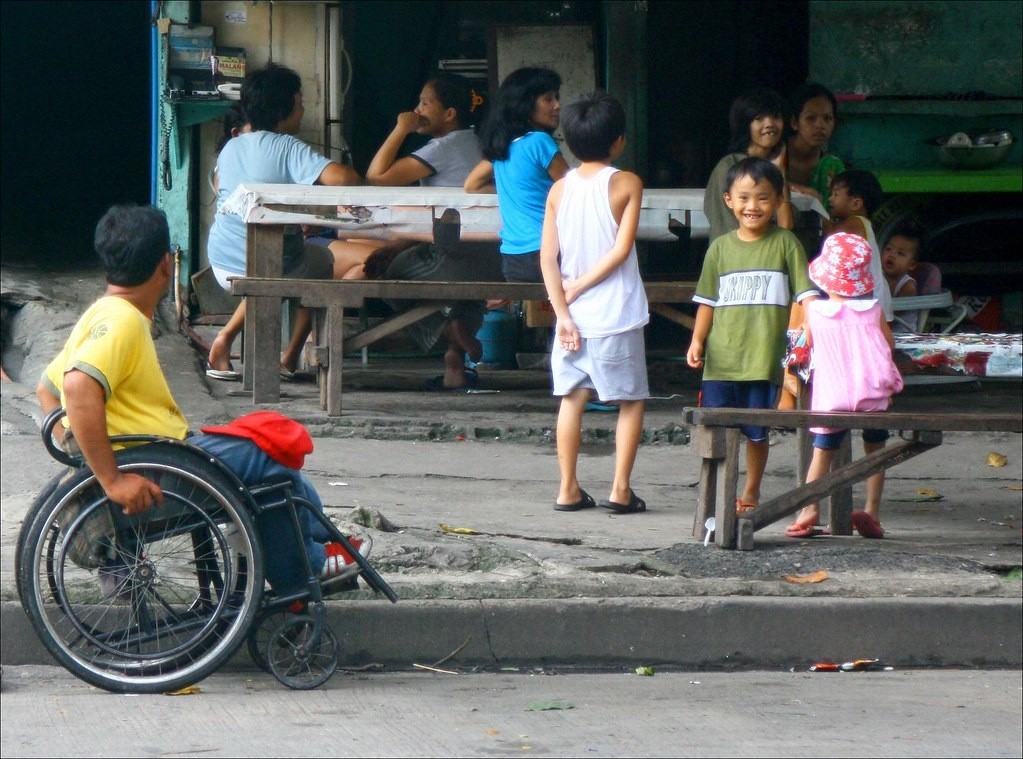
[199,411,315,471]
[808,231,875,298]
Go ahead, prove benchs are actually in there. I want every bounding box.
[679,404,1023,550]
[225,272,698,416]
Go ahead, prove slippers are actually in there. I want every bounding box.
[823,524,830,534]
[784,520,814,536]
[205,359,240,381]
[736,498,759,514]
[851,510,883,539]
[279,351,295,381]
[598,488,647,513]
[553,487,596,511]
[424,366,479,392]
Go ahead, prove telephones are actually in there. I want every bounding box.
[218,80,245,100]
[169,74,215,96]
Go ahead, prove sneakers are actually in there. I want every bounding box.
[288,532,374,613]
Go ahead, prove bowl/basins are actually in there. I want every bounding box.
[217,83,242,100]
[919,134,1018,170]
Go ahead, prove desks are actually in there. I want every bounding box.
[858,164,1023,279]
[216,180,831,405]
[780,325,1023,536]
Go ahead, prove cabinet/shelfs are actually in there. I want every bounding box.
[485,17,601,143]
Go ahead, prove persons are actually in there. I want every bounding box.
[699,84,926,428]
[541,90,650,511]
[787,232,904,535]
[207,61,399,381]
[37,206,371,611]
[688,154,820,514]
[465,65,570,283]
[349,68,490,392]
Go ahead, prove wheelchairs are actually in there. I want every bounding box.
[13,408,397,693]
